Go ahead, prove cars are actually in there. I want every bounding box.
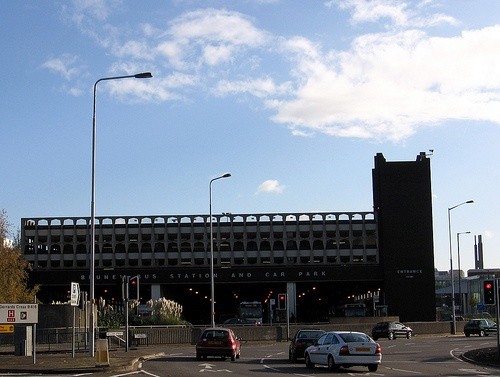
[195,329,241,361]
[464,319,497,336]
[304,331,382,372]
[289,330,326,362]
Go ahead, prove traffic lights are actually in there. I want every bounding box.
[278,293,286,310]
[128,277,139,301]
[483,280,496,305]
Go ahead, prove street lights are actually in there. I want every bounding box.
[448,200,474,335]
[209,173,231,335]
[90,72,153,359]
[457,232,471,319]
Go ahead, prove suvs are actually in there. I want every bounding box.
[372,321,413,340]
